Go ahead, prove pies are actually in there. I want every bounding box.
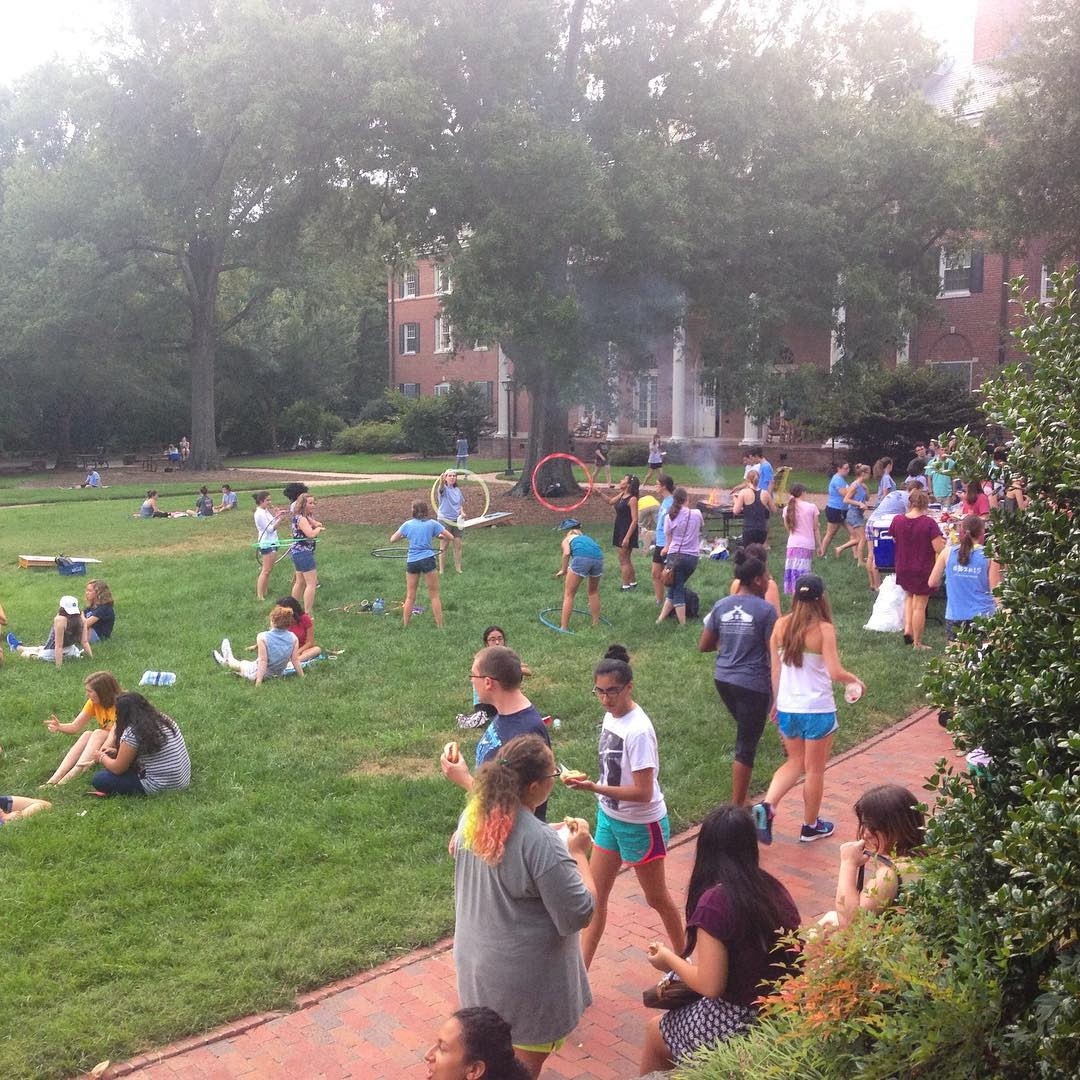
[563,816,577,832]
[648,942,663,955]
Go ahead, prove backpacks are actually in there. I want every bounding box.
[666,588,698,618]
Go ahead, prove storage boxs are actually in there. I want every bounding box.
[871,514,896,572]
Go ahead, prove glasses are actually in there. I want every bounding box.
[631,474,636,480]
[468,675,499,683]
[918,448,923,451]
[449,475,457,477]
[544,767,561,780]
[487,636,503,641]
[592,686,626,697]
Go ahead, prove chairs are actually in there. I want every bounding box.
[573,417,591,438]
[590,422,608,438]
[766,414,786,443]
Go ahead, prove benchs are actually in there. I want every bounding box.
[75,453,111,473]
[140,452,192,473]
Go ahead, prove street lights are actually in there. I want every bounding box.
[500,377,517,476]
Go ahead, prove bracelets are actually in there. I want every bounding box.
[100,753,107,764]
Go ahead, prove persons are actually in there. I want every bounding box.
[0,577,115,667]
[140,484,213,519]
[0,795,52,821]
[750,574,866,846]
[75,467,102,489]
[561,645,687,973]
[440,645,552,824]
[162,436,192,467]
[249,490,286,602]
[212,484,238,514]
[569,410,605,438]
[214,598,323,686]
[42,671,192,798]
[288,493,326,615]
[390,433,1071,1080]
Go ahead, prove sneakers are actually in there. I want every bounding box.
[800,816,834,842]
[752,801,775,845]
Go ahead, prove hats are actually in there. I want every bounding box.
[445,469,456,475]
[60,596,80,615]
[796,574,824,600]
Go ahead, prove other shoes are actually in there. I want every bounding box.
[7,634,22,653]
[40,783,65,792]
[542,715,552,726]
[913,646,931,650]
[213,650,226,665]
[904,634,913,644]
[620,582,637,592]
[222,639,233,659]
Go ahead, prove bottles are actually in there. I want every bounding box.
[554,718,561,730]
[844,682,862,703]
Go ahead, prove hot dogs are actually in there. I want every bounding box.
[444,741,458,763]
[559,770,587,785]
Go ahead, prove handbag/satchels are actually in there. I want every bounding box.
[458,710,488,729]
[642,973,702,1011]
[660,567,674,585]
